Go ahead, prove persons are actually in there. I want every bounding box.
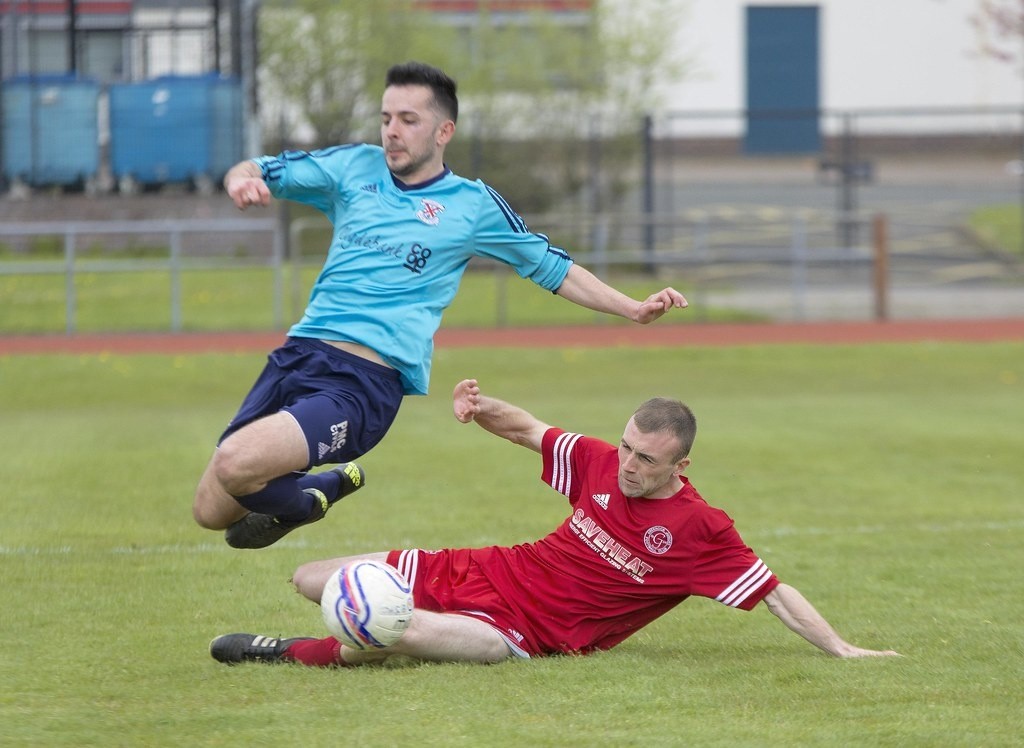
[192,60,691,549]
[208,378,905,669]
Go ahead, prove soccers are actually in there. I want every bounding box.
[319,559,415,654]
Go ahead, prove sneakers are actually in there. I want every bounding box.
[328,462,365,508]
[209,633,320,667]
[225,488,329,549]
